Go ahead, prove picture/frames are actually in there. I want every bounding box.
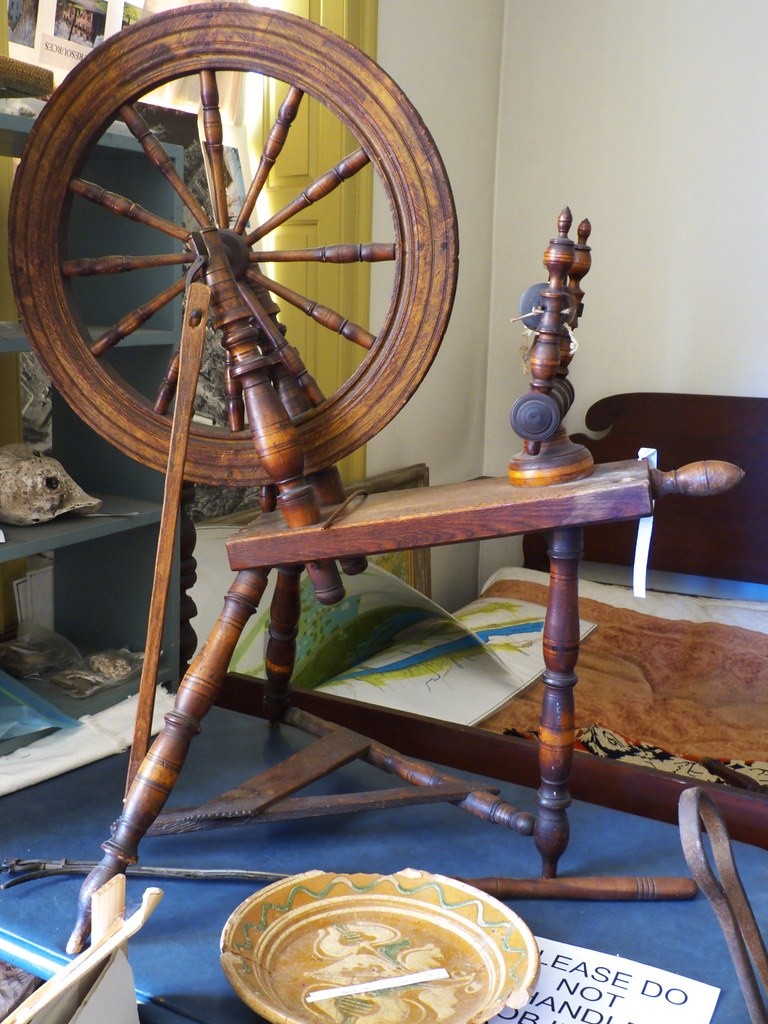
[343,465,439,599]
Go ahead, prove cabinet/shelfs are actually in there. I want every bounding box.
[0,115,195,756]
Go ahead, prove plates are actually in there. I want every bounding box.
[219,867,539,1024]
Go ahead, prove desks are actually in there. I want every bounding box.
[0,711,768,1024]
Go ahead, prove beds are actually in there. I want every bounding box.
[312,393,768,796]
